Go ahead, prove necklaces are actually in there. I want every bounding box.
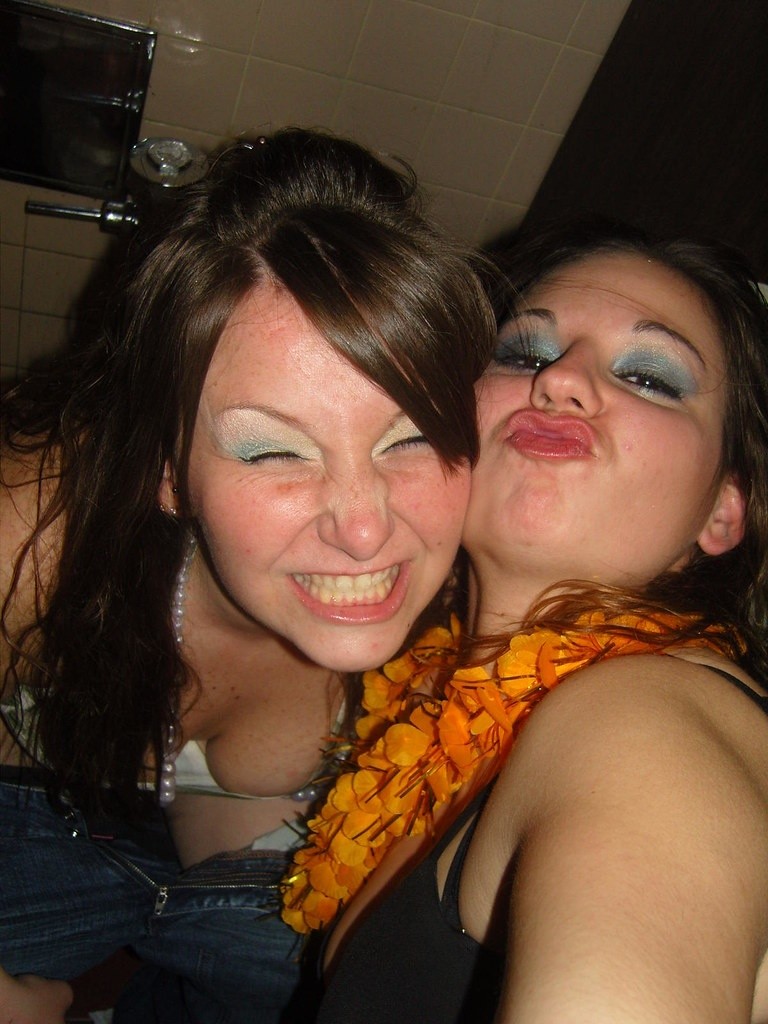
[158,537,350,804]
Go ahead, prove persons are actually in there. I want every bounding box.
[1,128,768,1024]
